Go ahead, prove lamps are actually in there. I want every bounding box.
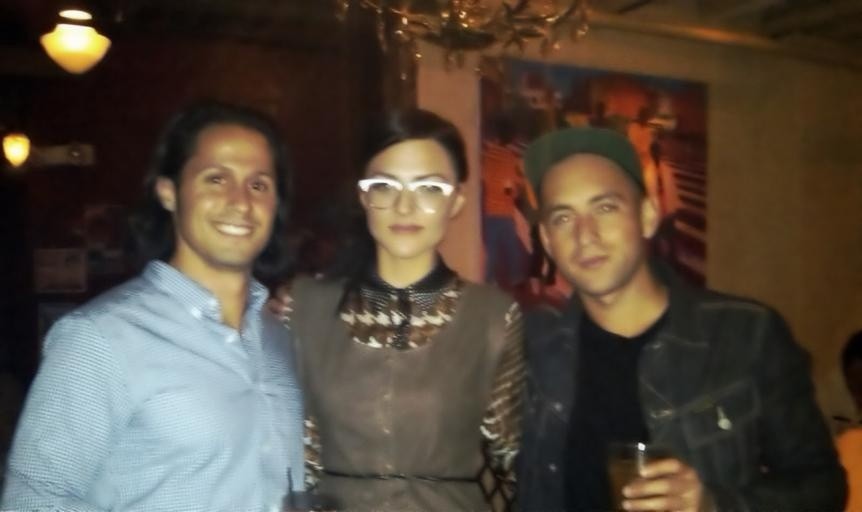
[38,22,112,75]
[335,0,592,82]
[2,132,102,175]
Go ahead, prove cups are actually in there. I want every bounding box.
[604,441,669,512]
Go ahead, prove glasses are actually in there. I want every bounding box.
[356,177,456,214]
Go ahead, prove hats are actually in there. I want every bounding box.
[524,126,645,201]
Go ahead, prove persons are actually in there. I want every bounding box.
[287,103,527,512]
[1,100,313,512]
[641,138,681,263]
[264,128,839,512]
[833,331,862,512]
